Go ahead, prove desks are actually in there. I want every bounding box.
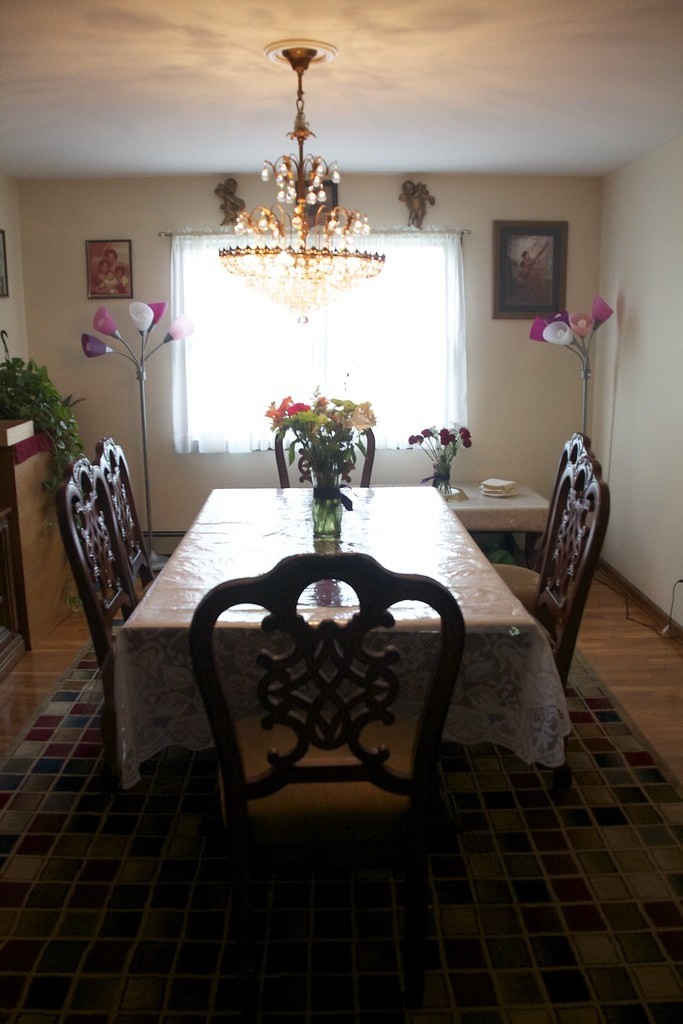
[432,481,552,567]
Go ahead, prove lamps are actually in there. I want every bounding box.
[527,292,615,432]
[211,36,386,322]
[77,298,195,573]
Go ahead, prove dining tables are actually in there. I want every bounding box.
[117,486,570,805]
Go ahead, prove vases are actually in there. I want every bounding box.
[308,462,349,538]
[430,459,455,496]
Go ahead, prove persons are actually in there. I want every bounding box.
[518,250,538,286]
[89,248,130,295]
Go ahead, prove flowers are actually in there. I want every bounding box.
[266,392,379,483]
[410,427,472,468]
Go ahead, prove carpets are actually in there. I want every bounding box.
[2,599,682,1024]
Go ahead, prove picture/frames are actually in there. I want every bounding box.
[489,218,571,323]
[84,237,135,301]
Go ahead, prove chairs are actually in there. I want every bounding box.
[55,435,160,679]
[488,435,614,657]
[184,548,470,997]
[273,412,378,490]
